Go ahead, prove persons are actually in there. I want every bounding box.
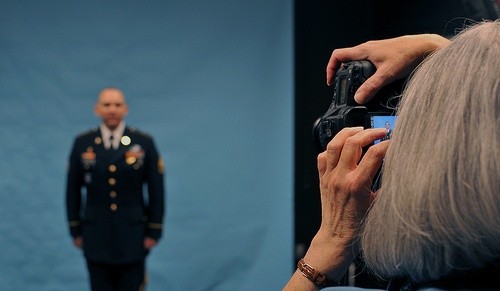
[65,87,165,291]
[281,21,500,291]
[380,121,393,141]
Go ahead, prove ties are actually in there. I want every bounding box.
[109,135,115,163]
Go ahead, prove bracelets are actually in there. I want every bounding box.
[297,258,328,290]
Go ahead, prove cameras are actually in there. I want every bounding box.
[313,60,399,166]
